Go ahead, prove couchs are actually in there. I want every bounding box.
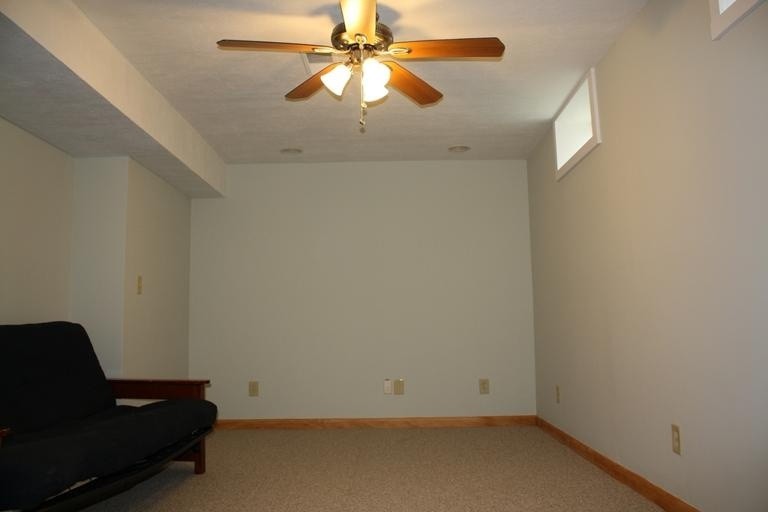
[0,319,219,512]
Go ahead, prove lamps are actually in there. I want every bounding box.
[319,44,392,109]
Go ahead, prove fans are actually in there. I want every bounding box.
[216,0,509,107]
